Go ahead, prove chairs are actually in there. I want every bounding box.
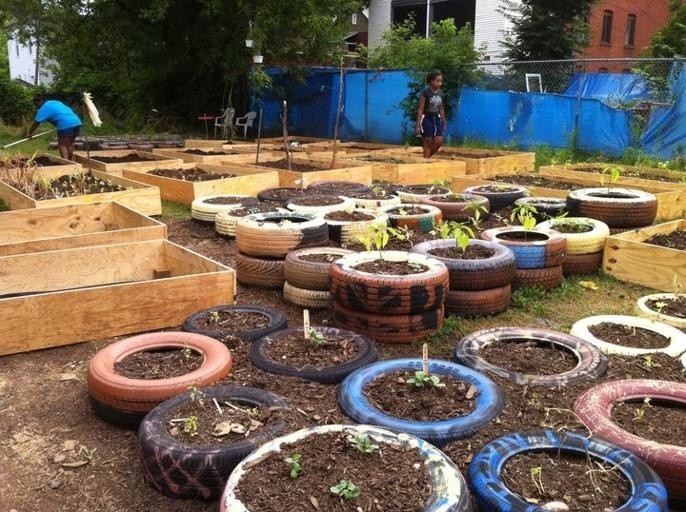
[213,107,257,139]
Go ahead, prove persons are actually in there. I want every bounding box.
[21,95,83,159]
[416,69,447,158]
